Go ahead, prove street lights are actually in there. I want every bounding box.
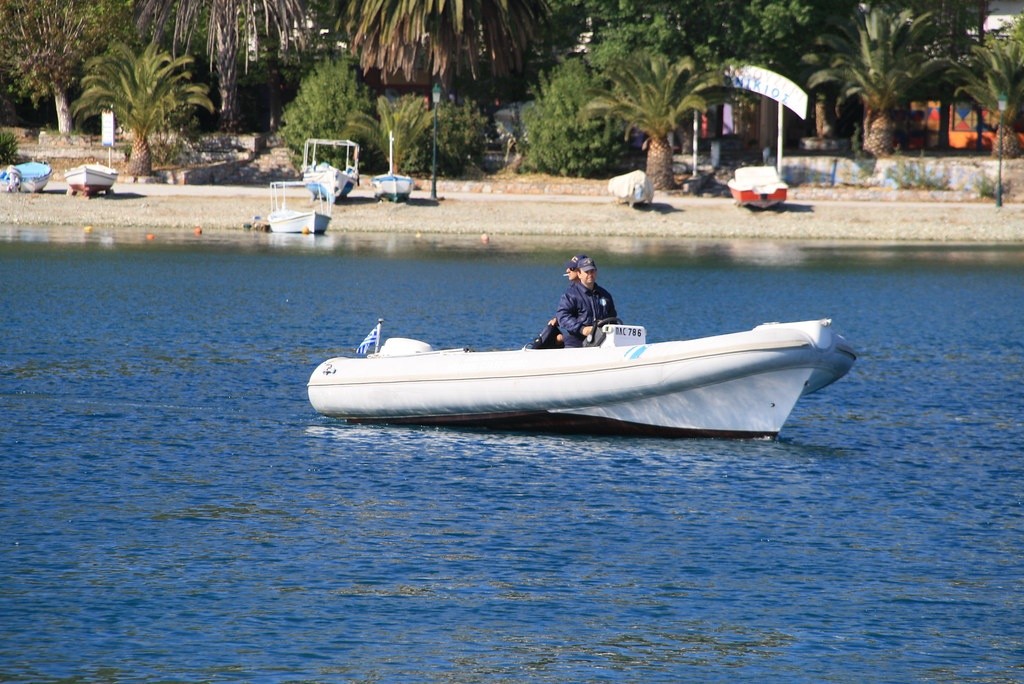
[430,83,441,200]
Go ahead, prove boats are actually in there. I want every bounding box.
[63,162,119,194]
[369,130,416,204]
[305,318,858,438]
[268,181,336,234]
[301,138,360,204]
[0,160,53,193]
[726,165,789,209]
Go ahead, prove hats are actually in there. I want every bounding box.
[566,255,588,268]
[576,257,597,272]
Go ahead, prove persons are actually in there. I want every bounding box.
[557,257,616,349]
[531,255,588,349]
